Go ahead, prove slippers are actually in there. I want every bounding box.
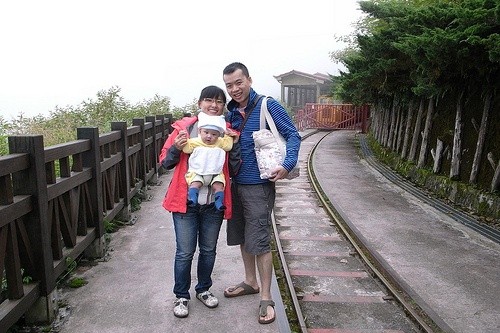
[258,300,276,324]
[224,281,260,298]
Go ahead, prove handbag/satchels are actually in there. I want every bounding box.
[253,96,300,180]
[227,143,242,176]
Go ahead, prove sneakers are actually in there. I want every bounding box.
[173,298,188,318]
[196,290,218,308]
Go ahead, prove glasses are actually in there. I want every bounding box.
[202,98,223,104]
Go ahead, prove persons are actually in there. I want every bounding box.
[159,85,241,319]
[178,112,233,211]
[222,62,302,325]
